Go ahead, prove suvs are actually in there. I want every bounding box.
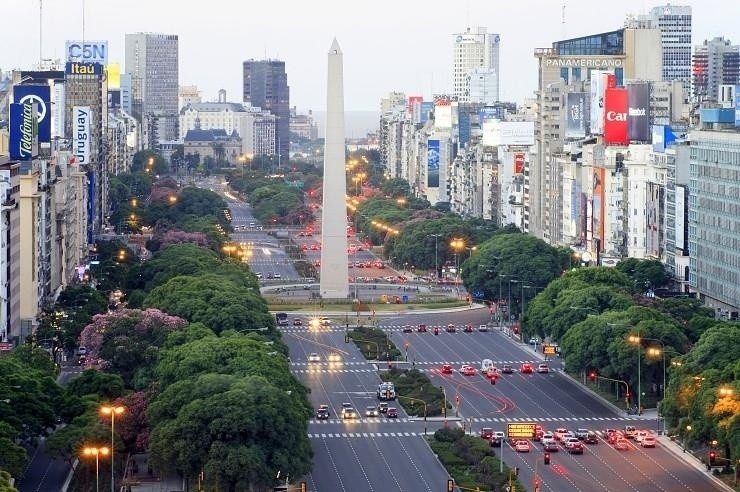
[574,428,588,439]
[387,407,397,418]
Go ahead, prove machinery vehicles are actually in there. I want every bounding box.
[377,381,396,400]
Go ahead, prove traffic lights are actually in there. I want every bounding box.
[710,451,715,464]
[515,466,519,475]
[201,471,206,481]
[536,480,539,487]
[544,452,550,465]
[442,407,446,414]
[491,375,495,384]
[440,385,444,393]
[511,485,515,492]
[456,395,459,404]
[590,371,594,381]
[435,328,438,335]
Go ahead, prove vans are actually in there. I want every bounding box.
[481,358,493,373]
[366,405,376,416]
[624,425,636,438]
[486,366,498,377]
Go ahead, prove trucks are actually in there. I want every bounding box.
[377,402,389,412]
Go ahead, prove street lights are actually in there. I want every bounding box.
[430,233,476,293]
[172,159,189,185]
[98,404,125,491]
[652,348,687,365]
[396,200,405,207]
[683,425,692,454]
[630,336,666,436]
[346,160,365,197]
[709,440,717,468]
[221,246,236,263]
[115,157,155,241]
[32,250,125,348]
[239,152,253,180]
[79,444,110,491]
[606,322,641,415]
[480,262,546,340]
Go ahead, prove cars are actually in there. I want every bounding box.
[481,426,506,448]
[232,222,263,232]
[342,402,355,418]
[583,432,599,444]
[292,224,321,267]
[459,364,474,375]
[346,225,431,285]
[603,428,628,451]
[305,203,319,211]
[77,346,87,366]
[532,425,557,453]
[633,430,648,442]
[501,364,512,373]
[509,438,529,452]
[274,312,330,327]
[251,271,280,280]
[308,351,340,362]
[315,404,329,419]
[521,363,532,372]
[537,363,548,373]
[641,436,655,448]
[362,178,388,199]
[402,324,473,332]
[441,364,452,373]
[529,338,539,345]
[553,427,583,454]
